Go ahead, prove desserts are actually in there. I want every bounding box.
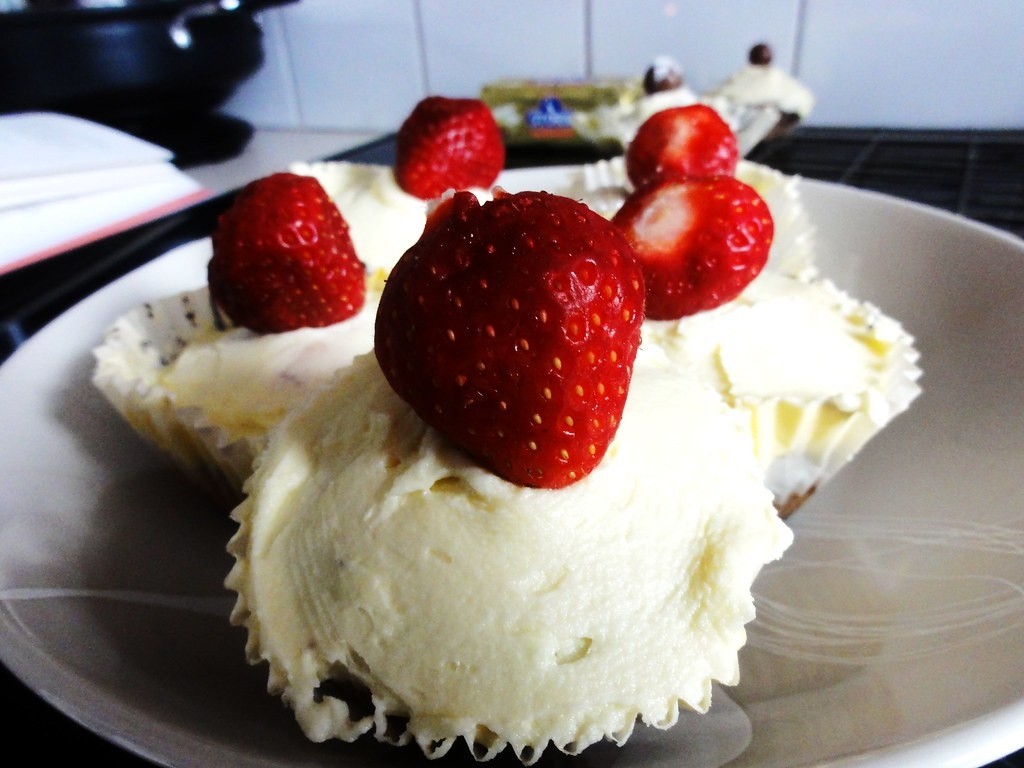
[614,60,709,152]
[227,344,791,761]
[304,161,501,278]
[723,43,815,138]
[642,270,919,516]
[91,272,379,479]
[580,158,810,281]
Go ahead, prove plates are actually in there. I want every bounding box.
[0,161,1024,768]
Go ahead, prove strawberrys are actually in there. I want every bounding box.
[628,108,737,191]
[614,175,774,317]
[396,94,504,199]
[208,173,365,333]
[372,195,644,489]
